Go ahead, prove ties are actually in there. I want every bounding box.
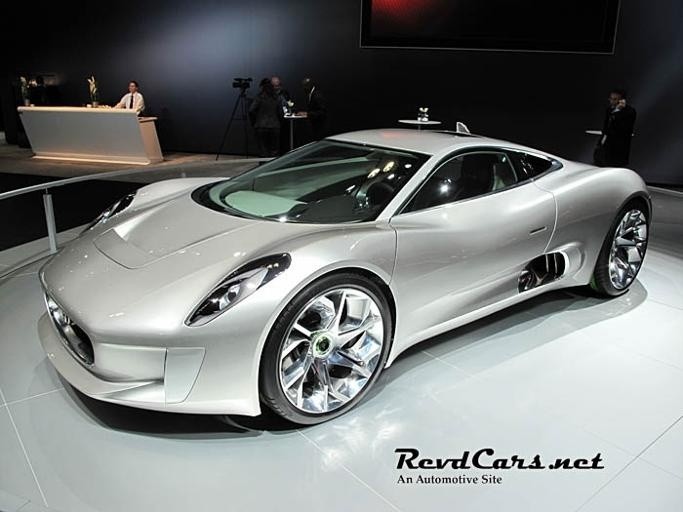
[130,96,133,108]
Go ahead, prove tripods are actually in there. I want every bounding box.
[216,93,254,160]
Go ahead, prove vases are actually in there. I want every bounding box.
[92,101,99,108]
[24,99,30,106]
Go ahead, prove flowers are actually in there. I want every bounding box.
[21,77,29,98]
[88,75,98,100]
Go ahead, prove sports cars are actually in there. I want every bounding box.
[34,119,654,429]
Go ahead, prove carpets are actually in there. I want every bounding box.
[1,173,148,254]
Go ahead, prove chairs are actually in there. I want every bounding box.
[365,182,396,210]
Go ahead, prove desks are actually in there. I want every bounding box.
[283,110,308,149]
[399,119,441,132]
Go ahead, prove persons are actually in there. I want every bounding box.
[247,75,331,157]
[113,81,145,118]
[593,88,637,169]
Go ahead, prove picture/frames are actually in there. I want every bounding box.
[360,0,621,54]
